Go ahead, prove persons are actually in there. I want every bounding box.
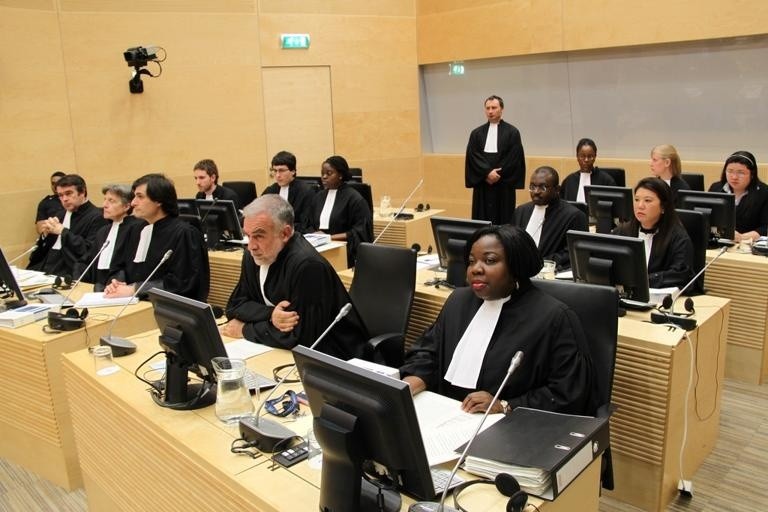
[507,166,591,273]
[105,173,210,301]
[297,156,374,245]
[29,174,111,278]
[193,159,240,218]
[610,176,698,295]
[464,95,526,222]
[708,151,768,243]
[650,143,693,189]
[260,152,312,210]
[219,194,373,364]
[33,171,65,232]
[73,181,143,281]
[398,222,607,421]
[561,137,610,211]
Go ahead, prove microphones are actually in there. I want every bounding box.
[99,249,173,356]
[527,216,549,238]
[410,350,524,512]
[238,302,352,453]
[650,246,728,330]
[372,205,406,244]
[45,240,110,331]
[397,179,424,214]
[6,245,38,266]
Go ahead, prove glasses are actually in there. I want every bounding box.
[271,168,292,175]
[724,170,753,181]
[527,183,557,194]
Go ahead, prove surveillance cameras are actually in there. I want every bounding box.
[124,46,166,94]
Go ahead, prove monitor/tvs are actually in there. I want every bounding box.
[148,287,232,411]
[0,248,28,309]
[195,199,244,252]
[676,189,735,250]
[566,230,650,317]
[297,176,324,193]
[430,216,492,288]
[292,344,436,512]
[176,198,202,232]
[584,185,634,233]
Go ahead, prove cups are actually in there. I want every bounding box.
[93,346,116,376]
[305,429,321,468]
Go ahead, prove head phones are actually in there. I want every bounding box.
[65,307,88,319]
[412,243,432,256]
[52,274,76,290]
[658,296,695,317]
[452,472,528,512]
[212,305,223,319]
[414,203,430,212]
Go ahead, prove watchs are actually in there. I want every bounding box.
[500,400,509,414]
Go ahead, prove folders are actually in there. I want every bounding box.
[649,287,679,305]
[304,233,331,247]
[454,407,611,503]
[0,302,51,329]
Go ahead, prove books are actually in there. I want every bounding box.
[301,234,330,248]
[0,304,60,329]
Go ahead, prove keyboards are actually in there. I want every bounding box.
[245,370,276,394]
[714,238,735,246]
[427,467,463,495]
[621,299,655,311]
[35,293,75,307]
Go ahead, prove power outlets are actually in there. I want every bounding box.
[677,480,694,498]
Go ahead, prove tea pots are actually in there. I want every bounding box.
[534,258,555,278]
[210,356,260,424]
[378,195,392,217]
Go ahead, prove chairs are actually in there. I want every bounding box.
[297,180,321,194]
[223,181,257,209]
[348,183,373,216]
[529,278,619,419]
[348,241,417,368]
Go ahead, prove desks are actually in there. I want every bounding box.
[373,207,446,253]
[337,251,732,512]
[61,314,604,512]
[207,240,348,315]
[0,270,159,492]
[703,243,768,386]
